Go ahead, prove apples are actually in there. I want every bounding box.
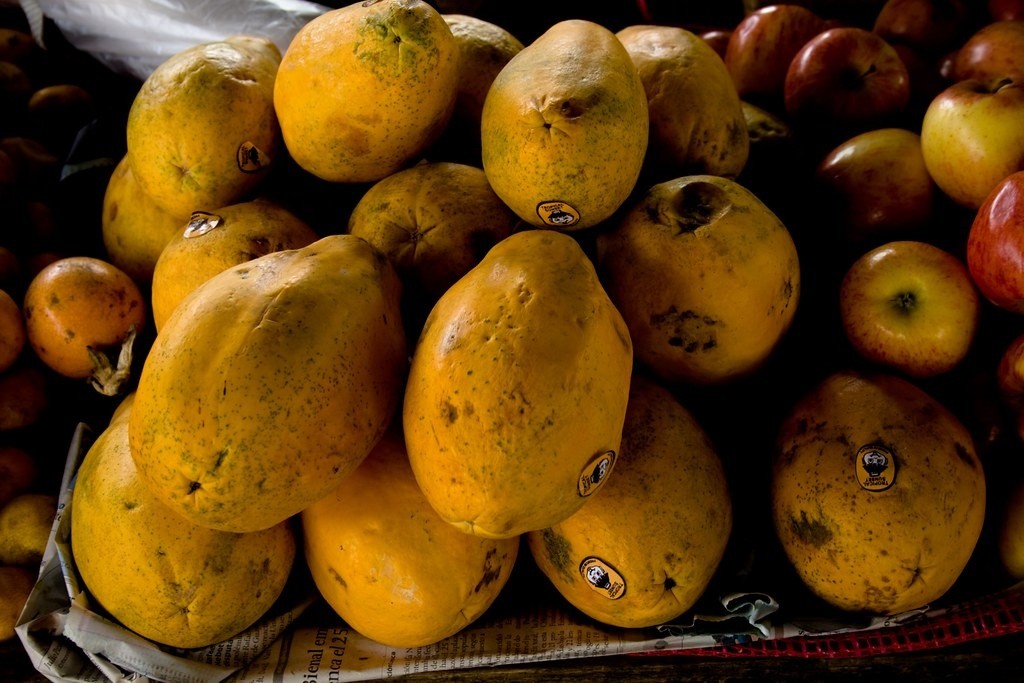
[689,0,1024,581]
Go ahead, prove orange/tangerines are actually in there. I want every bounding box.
[0,19,150,649]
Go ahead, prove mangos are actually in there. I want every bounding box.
[67,0,986,650]
